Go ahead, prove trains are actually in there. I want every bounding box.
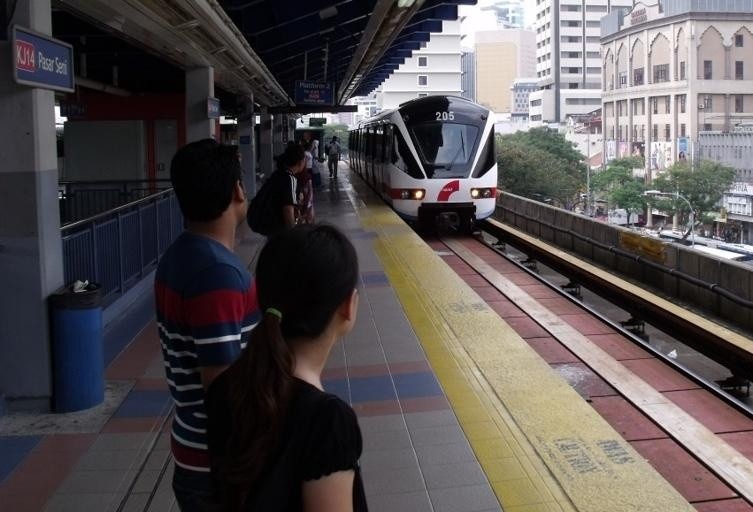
[348,94,500,230]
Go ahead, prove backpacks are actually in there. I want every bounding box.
[246,170,296,237]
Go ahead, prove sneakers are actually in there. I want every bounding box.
[329,172,337,180]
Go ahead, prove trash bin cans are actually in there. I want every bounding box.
[48,280,104,414]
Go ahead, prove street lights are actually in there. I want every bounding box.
[644,188,696,250]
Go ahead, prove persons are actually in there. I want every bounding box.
[248,134,342,240]
[152,139,262,511]
[206,223,368,512]
